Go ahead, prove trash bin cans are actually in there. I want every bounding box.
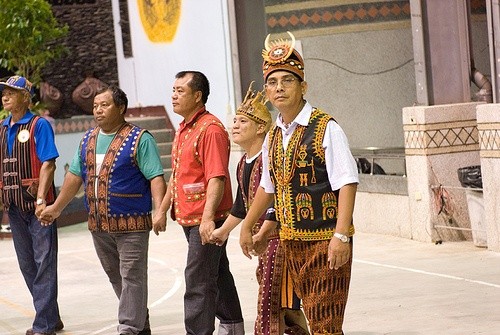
[458,165,488,247]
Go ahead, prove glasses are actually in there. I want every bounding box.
[266,78,300,88]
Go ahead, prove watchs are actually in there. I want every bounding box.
[334,232,350,243]
[37,199,43,206]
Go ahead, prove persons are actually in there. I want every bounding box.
[240,45,359,335]
[0,76,64,335]
[153,71,244,335]
[209,98,310,335]
[40,85,168,335]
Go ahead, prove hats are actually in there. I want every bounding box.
[0,77,33,96]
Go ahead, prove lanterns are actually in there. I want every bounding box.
[136,0,180,43]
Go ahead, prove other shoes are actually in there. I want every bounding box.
[27,320,64,335]
[138,328,151,335]
